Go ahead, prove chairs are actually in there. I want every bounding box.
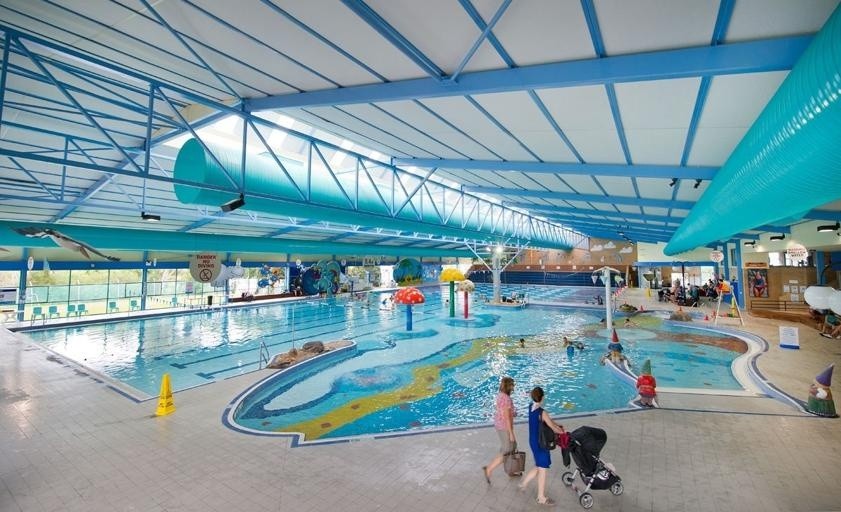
[30,299,140,325]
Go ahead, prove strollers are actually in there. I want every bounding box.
[562,426,623,509]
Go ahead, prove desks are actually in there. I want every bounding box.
[0,309,25,325]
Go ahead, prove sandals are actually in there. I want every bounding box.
[537,496,557,506]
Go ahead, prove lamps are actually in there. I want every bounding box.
[220,198,245,213]
[744,240,757,246]
[141,212,160,222]
[768,232,786,241]
[619,232,634,245]
[817,221,841,233]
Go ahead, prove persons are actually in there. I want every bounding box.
[809,308,829,324]
[519,337,526,348]
[751,269,767,297]
[624,318,638,328]
[819,315,841,338]
[657,276,724,305]
[562,336,572,347]
[481,375,523,486]
[515,386,565,507]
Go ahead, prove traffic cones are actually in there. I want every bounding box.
[647,287,651,298]
[156,372,175,416]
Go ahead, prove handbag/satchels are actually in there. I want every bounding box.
[508,472,523,476]
[538,420,556,450]
[503,451,527,472]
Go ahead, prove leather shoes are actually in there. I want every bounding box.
[482,466,493,485]
